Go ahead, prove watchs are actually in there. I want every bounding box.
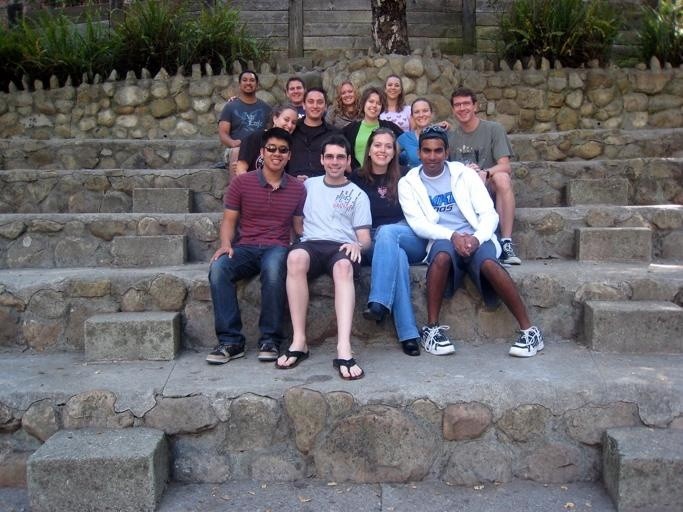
[358,242,365,248]
[481,168,490,179]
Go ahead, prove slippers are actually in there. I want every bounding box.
[275,349,309,369]
[333,358,365,380]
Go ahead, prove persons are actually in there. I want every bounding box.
[446,86,522,265]
[204,127,308,365]
[217,69,451,177]
[275,133,374,380]
[397,125,545,359]
[296,126,480,356]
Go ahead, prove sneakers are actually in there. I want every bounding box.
[206,344,245,364]
[257,344,278,361]
[499,240,522,264]
[420,325,455,355]
[509,325,544,357]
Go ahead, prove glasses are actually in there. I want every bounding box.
[419,126,444,134]
[325,154,346,161]
[264,145,290,153]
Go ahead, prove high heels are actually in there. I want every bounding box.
[402,338,420,355]
[363,302,389,326]
